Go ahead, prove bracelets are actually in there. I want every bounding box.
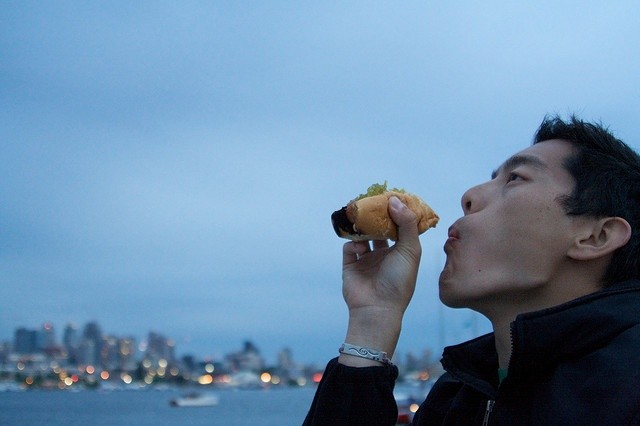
[338,341,395,366]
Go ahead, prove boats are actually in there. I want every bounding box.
[168,392,219,407]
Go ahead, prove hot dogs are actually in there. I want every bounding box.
[330,179,440,244]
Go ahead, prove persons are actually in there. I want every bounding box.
[302,113,640,426]
